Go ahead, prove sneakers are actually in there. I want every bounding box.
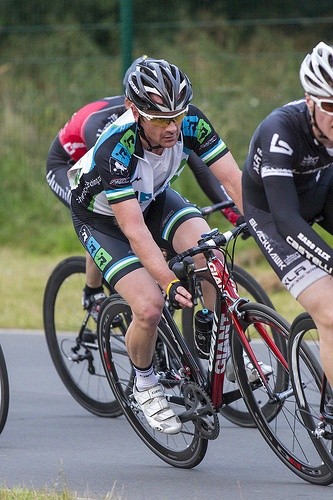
[133,376,183,435]
[320,392,333,418]
[152,347,175,389]
[82,291,123,329]
[225,350,274,383]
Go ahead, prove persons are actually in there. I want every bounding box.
[241,41,333,420]
[67,60,273,435]
[45,55,194,291]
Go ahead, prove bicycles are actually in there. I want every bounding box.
[42,198,333,485]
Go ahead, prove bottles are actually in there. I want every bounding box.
[194,309,214,359]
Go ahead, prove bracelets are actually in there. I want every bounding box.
[167,279,180,295]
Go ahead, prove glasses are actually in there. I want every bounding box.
[132,104,189,128]
[307,95,333,116]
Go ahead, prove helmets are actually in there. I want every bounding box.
[299,41,333,98]
[125,59,193,113]
[122,55,155,85]
